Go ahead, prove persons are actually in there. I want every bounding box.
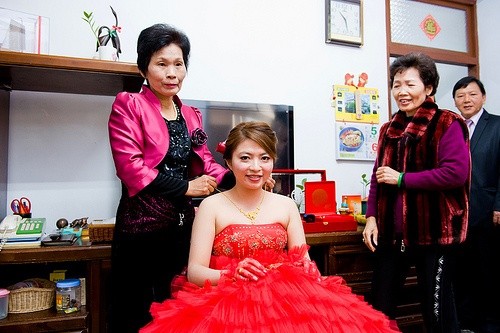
[363,52,500,333]
[143,120,401,333]
[104,25,277,332]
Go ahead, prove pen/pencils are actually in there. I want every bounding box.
[13,202,29,214]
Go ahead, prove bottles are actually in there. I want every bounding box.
[55,278,81,314]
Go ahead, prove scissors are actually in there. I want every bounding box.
[10,197,31,214]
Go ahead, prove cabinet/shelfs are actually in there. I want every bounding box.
[0,49,145,333]
[306,232,422,326]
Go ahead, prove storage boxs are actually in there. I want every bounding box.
[302,181,357,234]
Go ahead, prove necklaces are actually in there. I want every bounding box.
[215,183,268,226]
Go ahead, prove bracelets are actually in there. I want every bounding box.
[398,172,405,187]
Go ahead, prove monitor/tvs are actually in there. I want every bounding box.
[179,98,295,200]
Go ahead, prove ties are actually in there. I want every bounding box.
[465,119,473,139]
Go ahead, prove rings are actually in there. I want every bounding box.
[362,238,364,241]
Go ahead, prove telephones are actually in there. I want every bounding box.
[0,215,46,240]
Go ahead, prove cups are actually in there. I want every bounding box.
[13,212,32,219]
[0,288,10,319]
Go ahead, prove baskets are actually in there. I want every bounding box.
[7,277,56,313]
[88,219,115,242]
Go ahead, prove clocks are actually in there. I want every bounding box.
[325,0,364,47]
